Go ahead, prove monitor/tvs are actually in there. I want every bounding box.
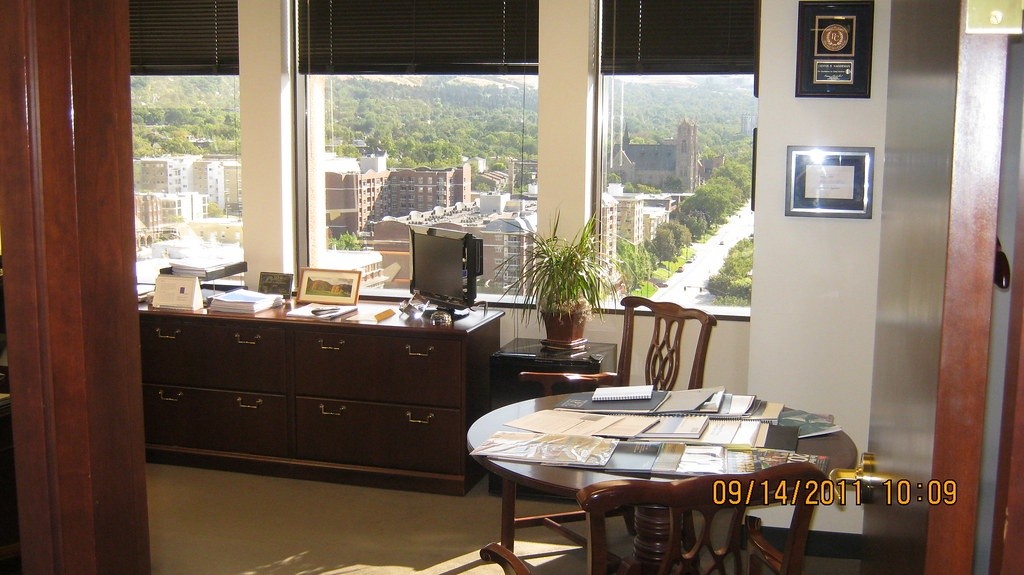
[408,223,483,321]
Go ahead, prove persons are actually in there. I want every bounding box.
[557,444,580,459]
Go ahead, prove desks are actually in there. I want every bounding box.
[137,289,505,495]
[464,388,858,575]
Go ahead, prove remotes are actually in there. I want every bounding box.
[312,307,339,315]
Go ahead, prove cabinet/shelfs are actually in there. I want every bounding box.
[488,337,618,497]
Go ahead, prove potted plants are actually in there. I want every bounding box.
[494,206,634,350]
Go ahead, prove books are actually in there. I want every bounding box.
[211,288,285,314]
[286,302,358,320]
[468,385,842,475]
[170,257,235,278]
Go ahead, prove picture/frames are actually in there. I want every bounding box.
[786,145,873,219]
[297,267,362,306]
[796,0,875,98]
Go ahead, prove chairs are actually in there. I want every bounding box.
[517,297,716,533]
[479,462,828,575]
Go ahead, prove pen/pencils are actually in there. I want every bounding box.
[311,307,342,316]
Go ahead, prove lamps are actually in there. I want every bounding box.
[966,0,1023,33]
